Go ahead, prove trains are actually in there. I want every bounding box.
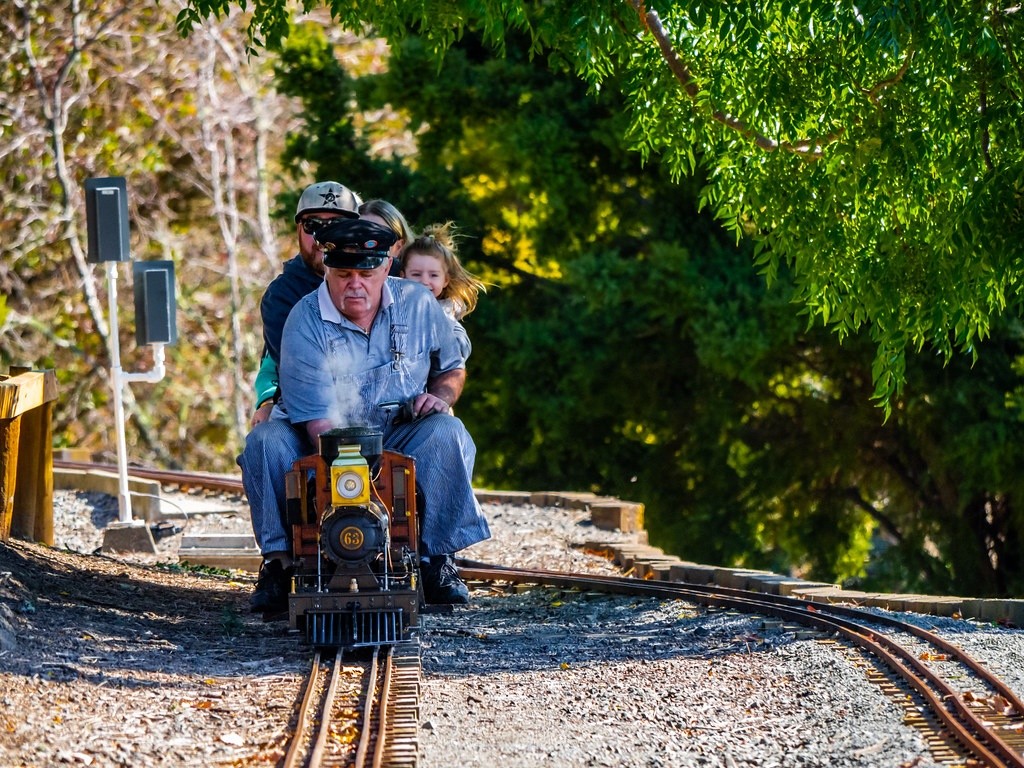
[286,426,420,648]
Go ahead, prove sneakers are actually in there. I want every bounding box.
[420,555,469,604]
[248,558,295,614]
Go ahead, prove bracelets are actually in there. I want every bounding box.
[261,401,273,406]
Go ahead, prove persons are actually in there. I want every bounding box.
[235,219,491,614]
[251,181,487,430]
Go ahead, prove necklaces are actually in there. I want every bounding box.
[345,309,377,335]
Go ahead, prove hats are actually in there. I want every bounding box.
[313,220,398,269]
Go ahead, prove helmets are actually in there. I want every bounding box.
[295,181,361,224]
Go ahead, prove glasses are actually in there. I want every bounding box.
[299,218,349,234]
